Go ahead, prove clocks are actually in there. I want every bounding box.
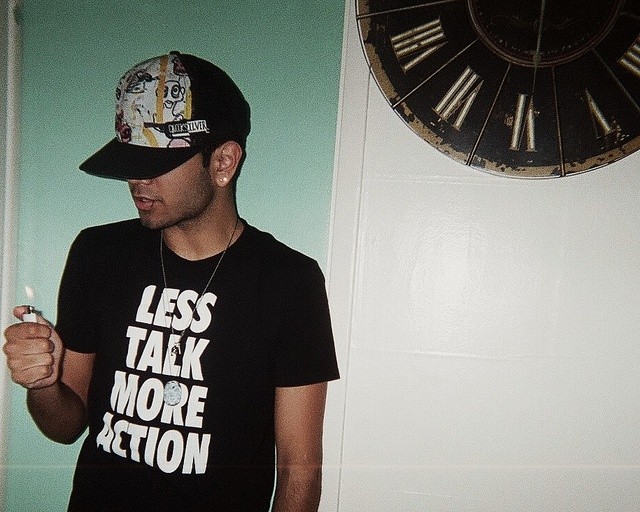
[354,1,640,179]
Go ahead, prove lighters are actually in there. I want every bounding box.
[22,306,37,323]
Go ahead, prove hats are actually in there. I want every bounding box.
[78,48,251,182]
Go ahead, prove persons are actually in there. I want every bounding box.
[3,51,340,512]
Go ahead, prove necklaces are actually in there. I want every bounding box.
[160,215,240,357]
[162,285,183,406]
